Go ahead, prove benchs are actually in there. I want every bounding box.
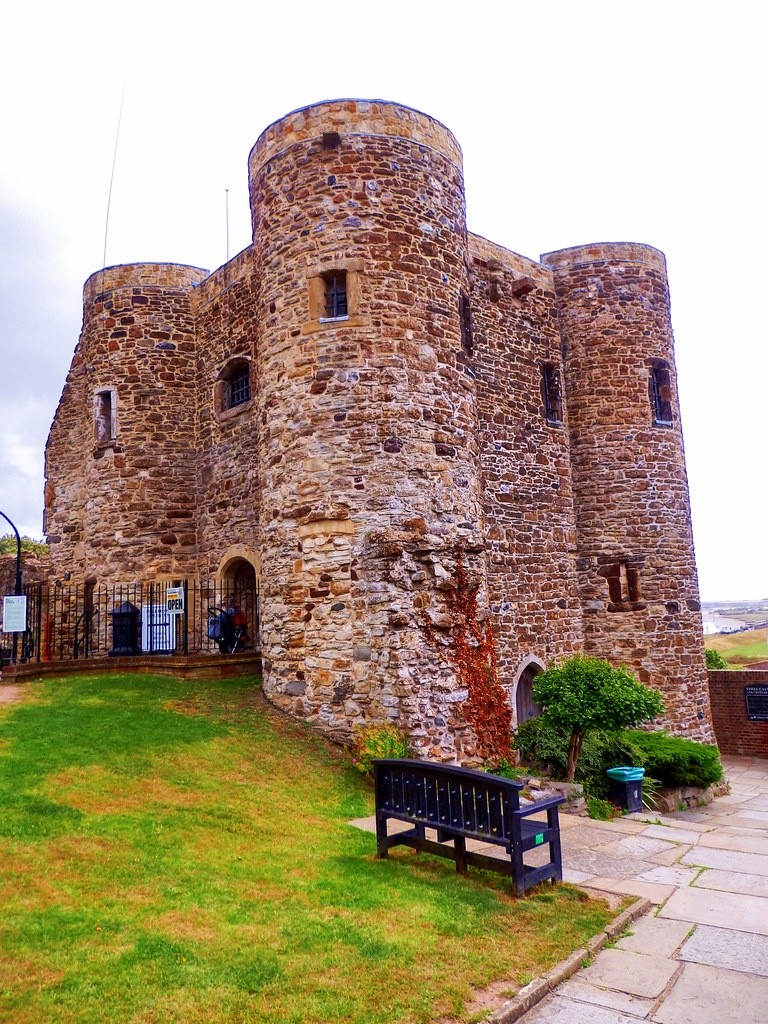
[370,759,566,899]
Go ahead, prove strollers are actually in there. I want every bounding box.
[205,607,248,656]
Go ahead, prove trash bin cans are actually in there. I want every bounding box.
[606,766,645,814]
